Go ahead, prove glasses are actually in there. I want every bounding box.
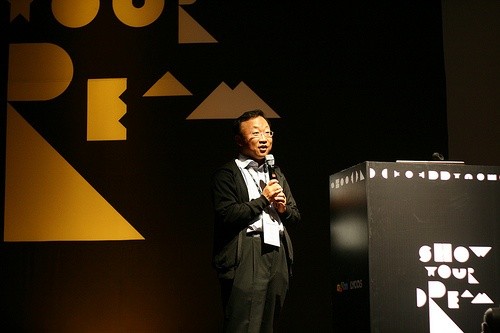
[251,130,274,137]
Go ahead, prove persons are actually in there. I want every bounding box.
[208,110,300,333]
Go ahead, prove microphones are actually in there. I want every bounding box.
[265,155,277,180]
[433,153,444,160]
